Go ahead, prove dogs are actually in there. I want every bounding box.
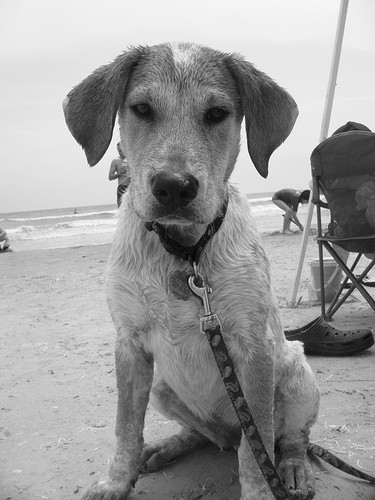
[61,41,322,500]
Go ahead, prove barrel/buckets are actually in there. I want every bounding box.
[310,257,342,303]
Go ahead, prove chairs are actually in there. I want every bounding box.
[310,130,375,322]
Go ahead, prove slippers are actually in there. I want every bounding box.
[337,272,355,289]
[280,314,375,355]
[356,272,375,286]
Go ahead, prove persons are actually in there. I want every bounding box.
[270,187,309,235]
[108,142,129,207]
